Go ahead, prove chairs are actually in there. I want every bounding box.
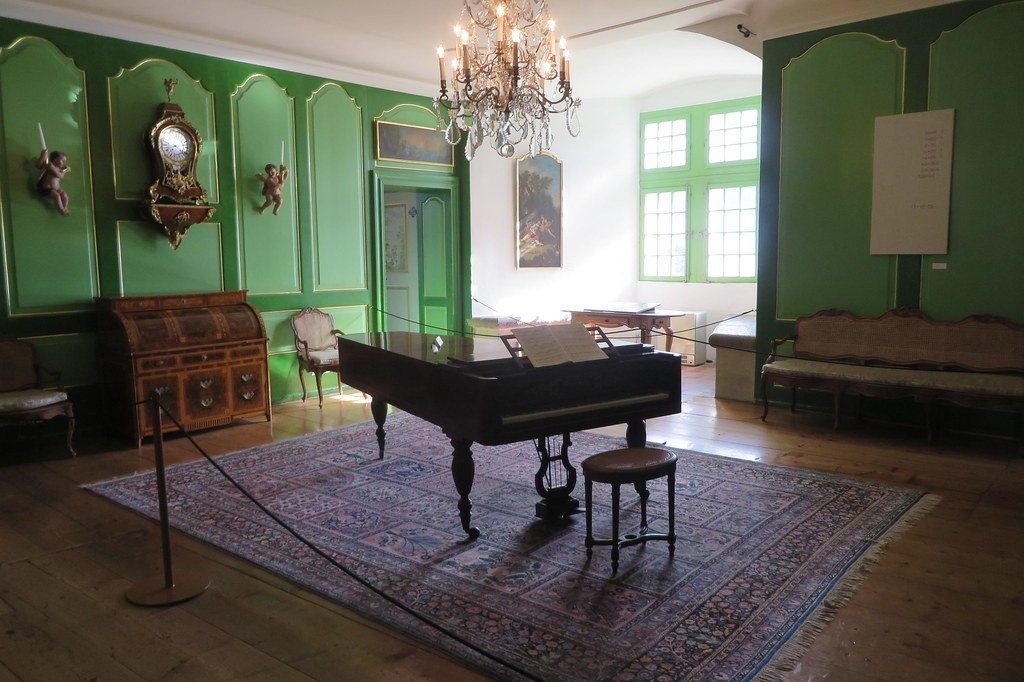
[290,306,366,409]
[1,337,77,458]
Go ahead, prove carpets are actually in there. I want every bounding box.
[78,411,944,682]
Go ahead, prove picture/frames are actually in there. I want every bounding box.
[515,150,564,271]
[386,286,410,332]
[385,203,409,273]
[376,119,455,168]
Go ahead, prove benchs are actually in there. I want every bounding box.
[708,313,756,403]
[760,308,1024,443]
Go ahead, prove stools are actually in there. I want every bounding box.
[581,447,678,577]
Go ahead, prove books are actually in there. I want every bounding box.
[510,323,610,369]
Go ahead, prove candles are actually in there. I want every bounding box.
[281,140,284,165]
[38,122,47,150]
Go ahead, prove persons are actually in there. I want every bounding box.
[257,163,289,216]
[35,148,72,216]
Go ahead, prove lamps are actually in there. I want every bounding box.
[436,0,582,161]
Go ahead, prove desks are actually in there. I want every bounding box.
[559,309,687,353]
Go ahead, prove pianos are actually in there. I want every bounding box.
[337,331,684,538]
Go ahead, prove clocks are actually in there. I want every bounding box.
[143,103,210,207]
